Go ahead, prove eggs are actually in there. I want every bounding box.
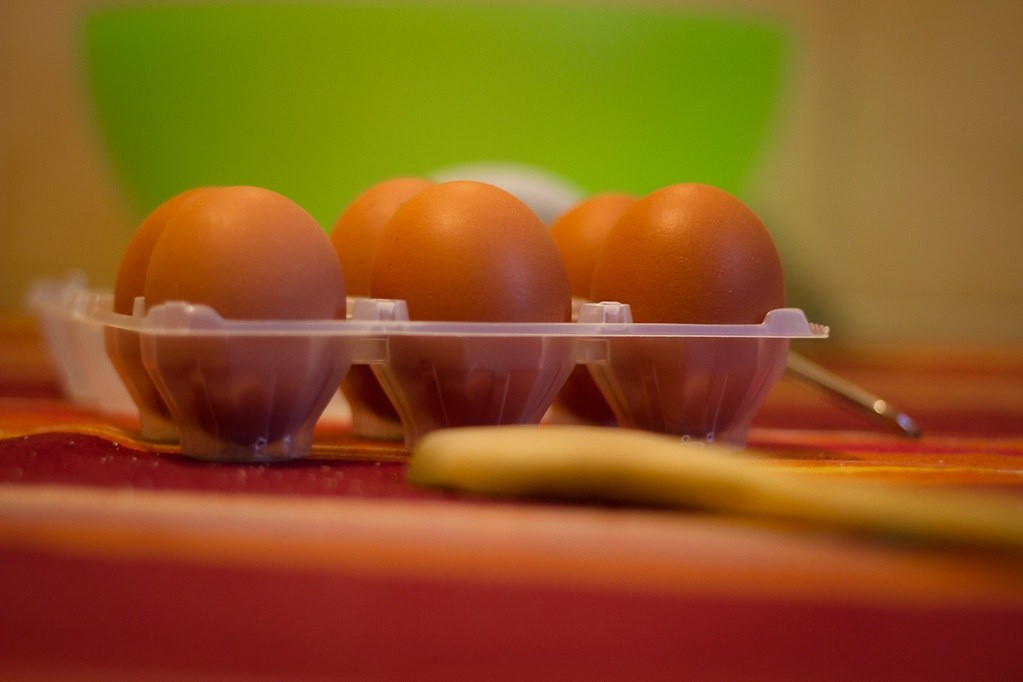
[105,174,784,442]
